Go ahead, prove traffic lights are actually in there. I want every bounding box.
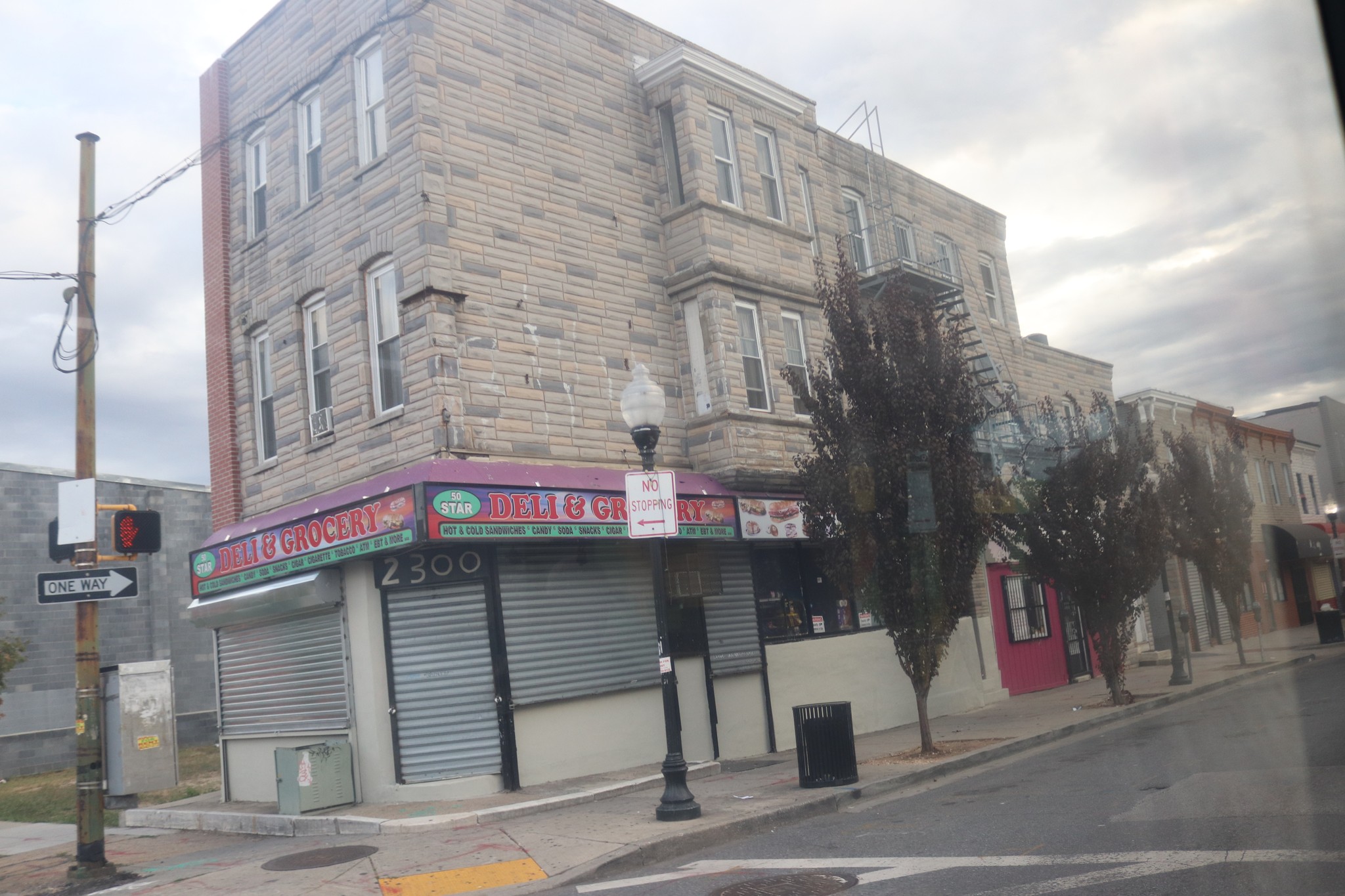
[114,509,161,556]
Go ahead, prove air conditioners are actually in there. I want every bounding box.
[308,405,334,438]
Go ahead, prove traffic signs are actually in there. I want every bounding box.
[625,470,679,539]
[35,567,139,606]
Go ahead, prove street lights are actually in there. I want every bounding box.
[1324,493,1345,604]
[619,361,703,821]
[1140,462,1191,685]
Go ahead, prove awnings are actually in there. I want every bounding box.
[1262,521,1334,563]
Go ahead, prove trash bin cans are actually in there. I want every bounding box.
[1315,610,1345,643]
[792,701,859,788]
